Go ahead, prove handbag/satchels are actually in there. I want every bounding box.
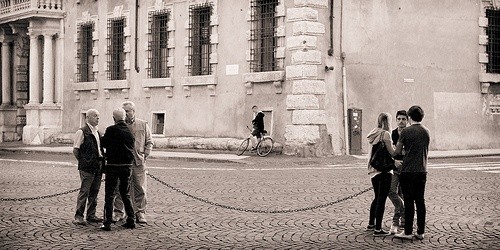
[369,130,396,173]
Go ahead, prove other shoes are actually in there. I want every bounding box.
[136,212,147,223]
[74,216,87,226]
[391,223,398,232]
[400,222,404,230]
[251,147,257,151]
[395,231,412,239]
[99,225,112,231]
[112,209,126,221]
[414,231,425,239]
[373,229,391,236]
[366,223,377,230]
[86,216,104,222]
[121,220,136,229]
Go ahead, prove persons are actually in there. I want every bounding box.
[100,101,154,231]
[367,105,430,239]
[73,108,106,225]
[250,106,265,156]
